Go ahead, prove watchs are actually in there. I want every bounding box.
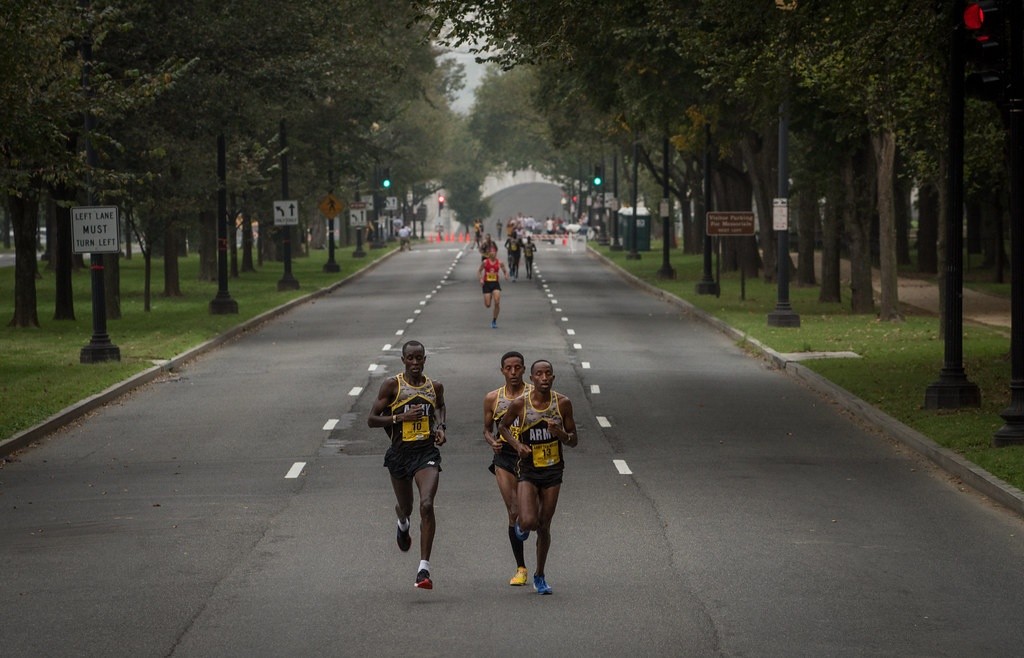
[436,423,446,431]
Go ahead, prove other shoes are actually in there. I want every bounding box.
[492,321,497,328]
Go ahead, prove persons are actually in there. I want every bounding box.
[479,233,498,261]
[392,216,412,251]
[481,352,535,587]
[544,211,589,246]
[478,250,512,330]
[506,211,543,240]
[524,236,536,279]
[470,218,484,249]
[504,231,525,283]
[366,340,447,590]
[498,359,578,595]
[497,218,503,240]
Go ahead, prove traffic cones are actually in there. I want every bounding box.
[430,230,471,243]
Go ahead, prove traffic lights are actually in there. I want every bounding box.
[439,195,445,209]
[572,193,579,203]
[380,165,392,189]
[950,0,987,34]
[593,166,603,189]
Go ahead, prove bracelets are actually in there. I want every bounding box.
[515,441,520,451]
[392,414,396,424]
[563,437,570,445]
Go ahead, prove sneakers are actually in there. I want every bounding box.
[397,516,411,551]
[510,566,527,585]
[515,516,530,541]
[533,572,552,595]
[414,568,432,589]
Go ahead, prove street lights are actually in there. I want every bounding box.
[767,0,801,327]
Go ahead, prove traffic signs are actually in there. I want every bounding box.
[273,200,299,226]
